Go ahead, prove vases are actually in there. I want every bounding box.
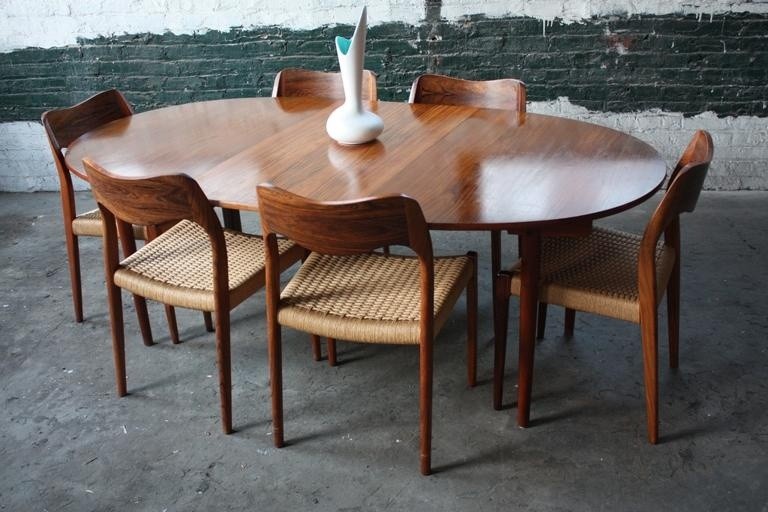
[327,6,385,148]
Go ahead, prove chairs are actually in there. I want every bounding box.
[81,156,322,437]
[493,130,713,444]
[271,69,528,259]
[255,181,479,476]
[42,89,222,344]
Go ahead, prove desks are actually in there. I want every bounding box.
[65,98,666,413]
